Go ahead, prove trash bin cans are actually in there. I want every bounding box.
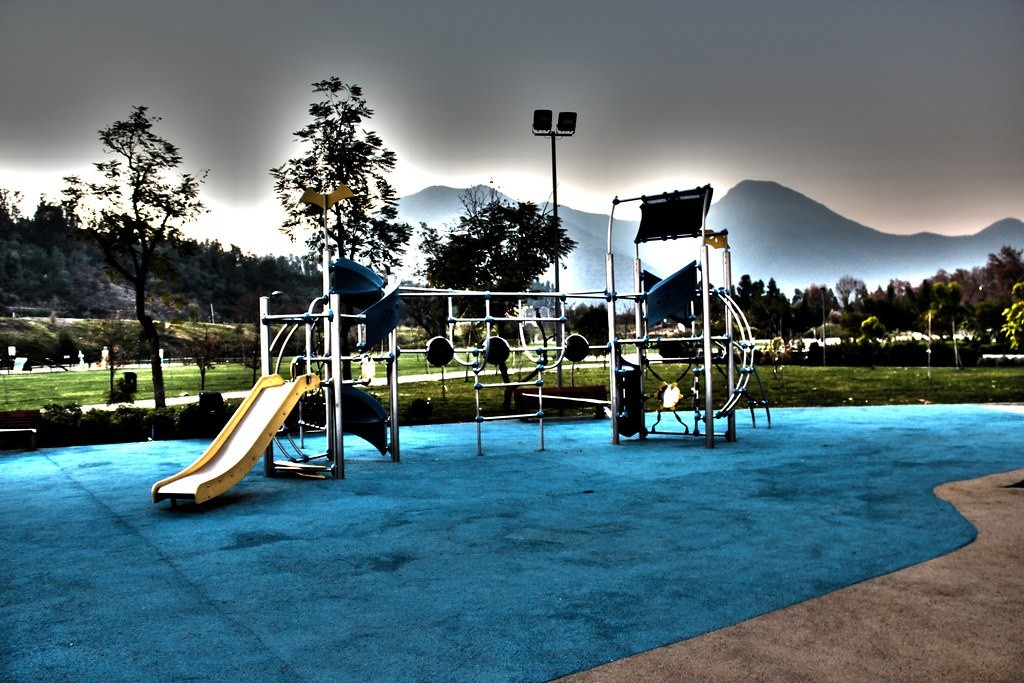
[199,391,224,407]
[124,372,137,393]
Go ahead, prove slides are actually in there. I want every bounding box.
[151,373,323,504]
